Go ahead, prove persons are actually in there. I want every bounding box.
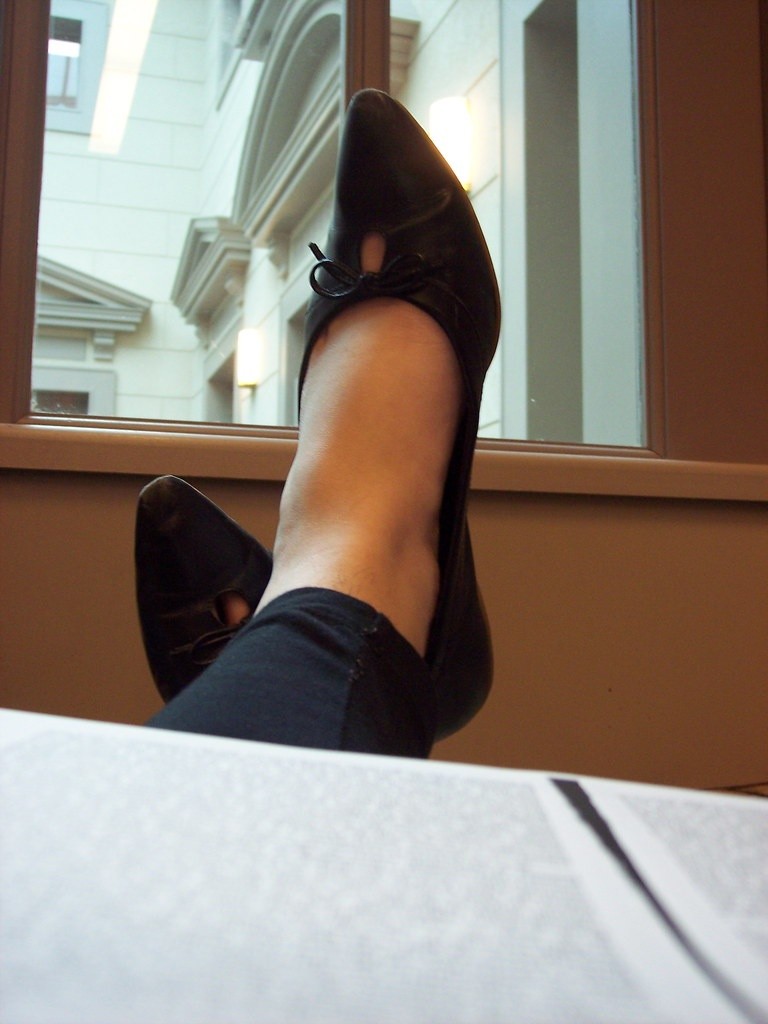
[133,89,504,759]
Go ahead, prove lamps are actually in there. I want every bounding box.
[428,95,475,190]
[235,328,257,388]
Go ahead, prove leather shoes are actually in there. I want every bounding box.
[295,88,495,743]
[134,474,274,704]
